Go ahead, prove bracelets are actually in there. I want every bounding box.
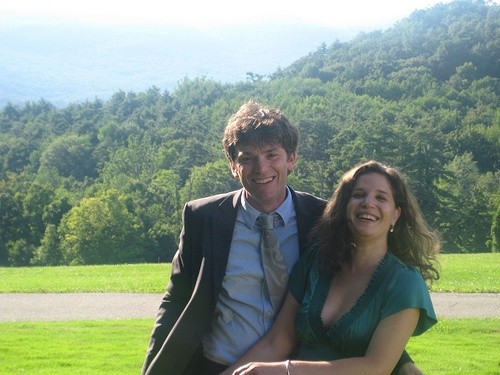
[285,359,291,375]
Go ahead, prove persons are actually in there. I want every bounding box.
[140,101,331,375]
[219,160,443,375]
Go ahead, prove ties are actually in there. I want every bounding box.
[259,213,290,319]
